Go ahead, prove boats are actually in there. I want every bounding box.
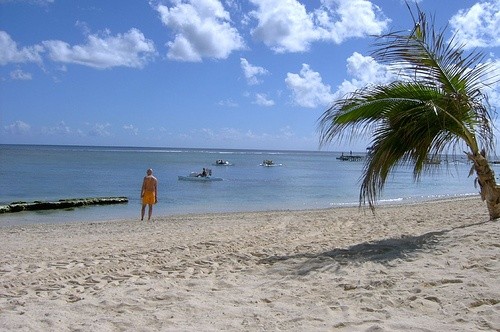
[216,159,233,166]
[336,150,365,162]
[178,172,224,182]
[260,161,283,167]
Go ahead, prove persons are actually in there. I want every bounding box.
[140,168,158,220]
[195,168,206,177]
